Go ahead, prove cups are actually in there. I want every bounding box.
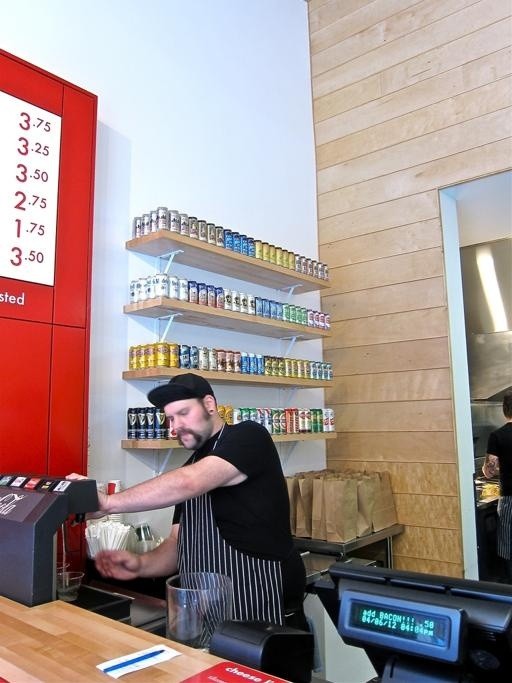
[55,559,69,571]
[127,521,161,555]
[58,572,84,602]
[96,480,122,496]
[86,514,123,525]
[166,573,231,651]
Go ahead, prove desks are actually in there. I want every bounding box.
[291,523,405,590]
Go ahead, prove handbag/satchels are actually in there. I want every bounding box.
[286,469,398,545]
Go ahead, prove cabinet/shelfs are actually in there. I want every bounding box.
[121,230,338,449]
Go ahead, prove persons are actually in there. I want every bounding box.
[59,371,311,632]
[482,390,511,585]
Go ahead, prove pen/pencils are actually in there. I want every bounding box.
[103,650,166,674]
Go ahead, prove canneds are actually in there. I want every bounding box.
[133,208,328,283]
[128,342,334,382]
[130,274,331,332]
[213,405,336,434]
[126,404,178,440]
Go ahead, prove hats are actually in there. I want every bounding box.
[146,372,215,410]
